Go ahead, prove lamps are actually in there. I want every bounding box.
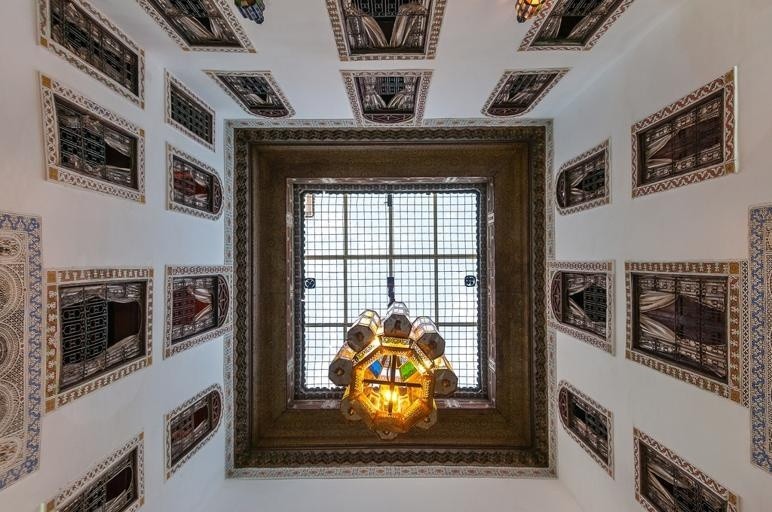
[326,303,458,441]
